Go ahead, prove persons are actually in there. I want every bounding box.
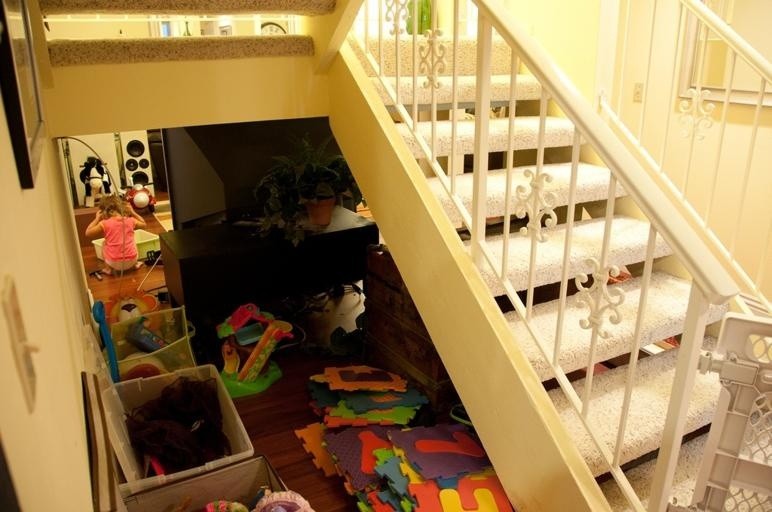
[85,195,148,276]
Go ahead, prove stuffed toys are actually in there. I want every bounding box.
[104,290,158,333]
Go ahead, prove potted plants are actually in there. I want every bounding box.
[251,139,365,226]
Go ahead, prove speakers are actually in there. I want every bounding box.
[118,131,156,198]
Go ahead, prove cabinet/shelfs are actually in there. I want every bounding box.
[158,203,378,334]
[357,238,457,411]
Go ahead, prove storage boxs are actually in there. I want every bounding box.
[106,306,302,512]
